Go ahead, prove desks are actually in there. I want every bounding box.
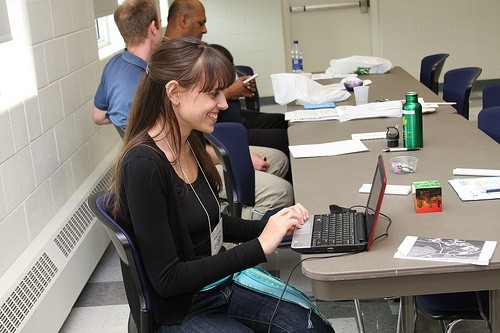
[286,113,500,333]
[285,66,459,126]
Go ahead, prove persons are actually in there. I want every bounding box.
[92,0,294,220]
[101,36,336,333]
[161,0,289,146]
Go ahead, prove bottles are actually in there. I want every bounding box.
[401,91,424,150]
[290,40,304,73]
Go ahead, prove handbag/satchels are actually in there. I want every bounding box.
[326,56,394,75]
[271,72,351,107]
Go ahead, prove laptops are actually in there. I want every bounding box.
[290,154,387,254]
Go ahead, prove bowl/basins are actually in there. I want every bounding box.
[389,156,419,174]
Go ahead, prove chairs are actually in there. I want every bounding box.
[86,52,500,333]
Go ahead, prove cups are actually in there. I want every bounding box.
[352,86,369,106]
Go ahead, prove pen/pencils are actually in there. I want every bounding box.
[486,188,500,193]
[382,148,423,152]
[264,157,266,161]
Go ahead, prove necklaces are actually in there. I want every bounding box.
[163,34,171,41]
